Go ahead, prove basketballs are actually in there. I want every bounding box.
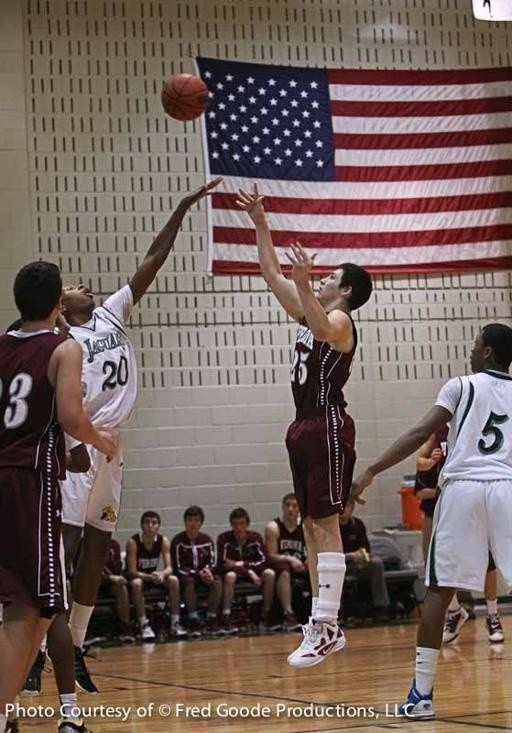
[161,73,208,120]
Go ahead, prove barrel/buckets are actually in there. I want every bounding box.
[397,487,427,530]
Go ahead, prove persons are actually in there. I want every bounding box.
[13,493,391,655]
[237,183,371,669]
[416,421,505,643]
[349,324,512,721]
[413,465,440,568]
[0,261,117,733]
[21,176,222,695]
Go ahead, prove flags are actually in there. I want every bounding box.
[197,57,512,275]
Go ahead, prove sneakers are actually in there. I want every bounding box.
[259,615,283,631]
[82,628,107,652]
[203,616,225,635]
[58,717,92,732]
[4,720,19,732]
[187,618,203,637]
[485,611,505,642]
[289,619,346,667]
[399,679,435,720]
[283,611,302,630]
[442,604,468,643]
[169,623,187,635]
[141,620,155,638]
[114,623,136,642]
[222,614,238,633]
[46,640,99,694]
[20,649,52,694]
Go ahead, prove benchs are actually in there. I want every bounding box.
[84,537,418,642]
